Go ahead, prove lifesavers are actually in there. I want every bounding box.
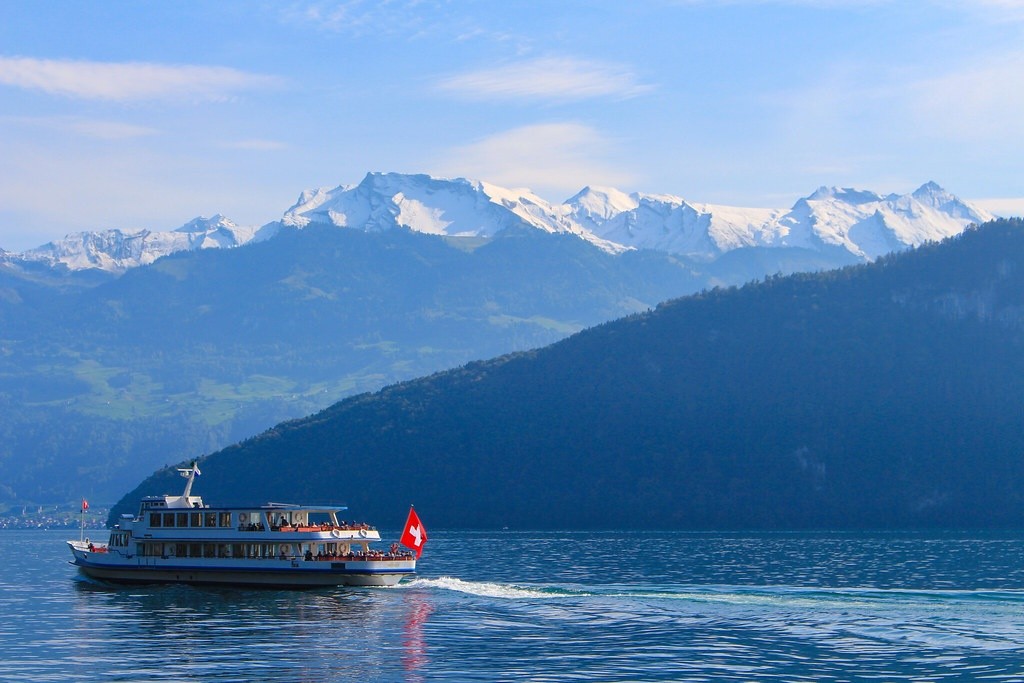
[295,512,303,522]
[238,512,247,522]
[359,529,368,537]
[339,543,347,553]
[280,544,289,554]
[331,529,341,538]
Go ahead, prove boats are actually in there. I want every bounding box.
[67,457,416,588]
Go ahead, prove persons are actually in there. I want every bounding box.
[241,523,257,530]
[281,516,288,526]
[88,543,95,552]
[304,551,313,561]
[322,521,334,526]
[317,542,412,557]
[340,521,364,527]
[312,522,317,527]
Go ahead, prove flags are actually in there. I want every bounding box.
[84,501,88,509]
[400,509,427,559]
